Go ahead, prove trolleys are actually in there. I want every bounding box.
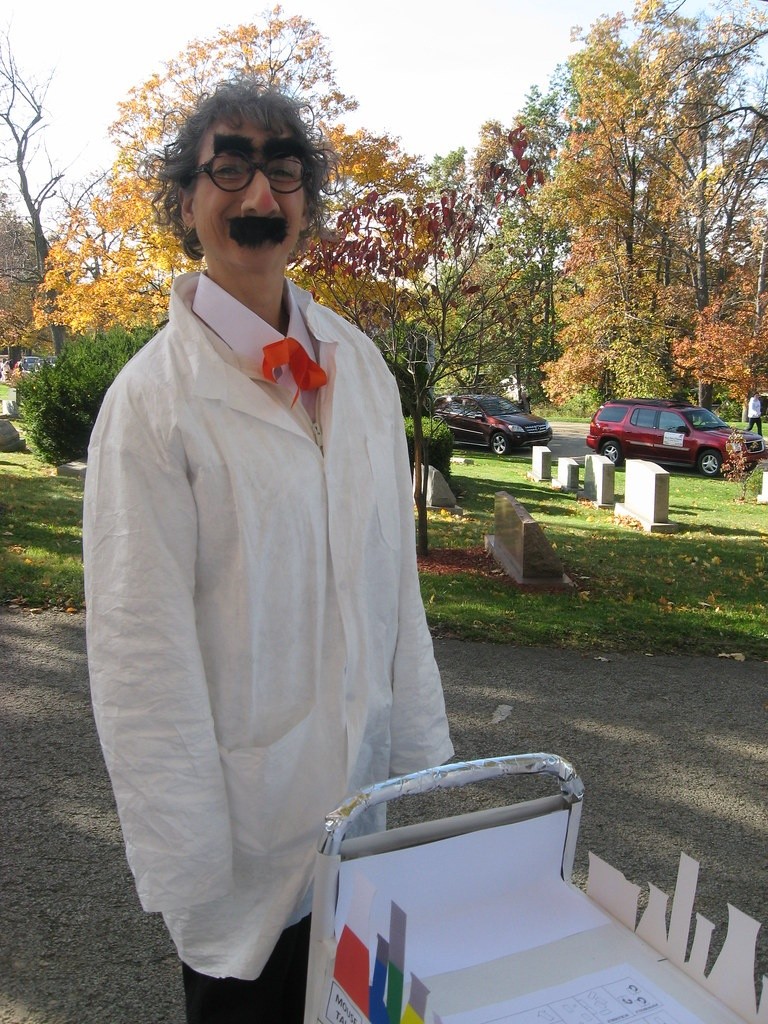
[305,748,768,1024]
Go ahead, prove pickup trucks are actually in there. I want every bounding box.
[586,396,766,477]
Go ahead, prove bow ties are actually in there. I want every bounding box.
[263,337,328,410]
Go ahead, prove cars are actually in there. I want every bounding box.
[44,356,56,367]
[21,355,42,370]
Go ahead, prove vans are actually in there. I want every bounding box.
[433,394,554,454]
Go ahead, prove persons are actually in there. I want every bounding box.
[83,78,455,1024]
[745,392,762,435]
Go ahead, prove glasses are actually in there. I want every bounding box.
[181,149,309,194]
